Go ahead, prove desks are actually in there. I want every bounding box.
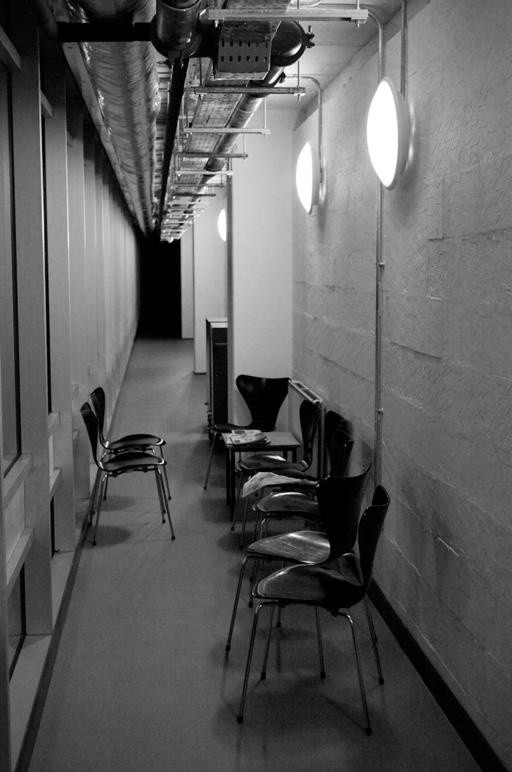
[221,429,302,518]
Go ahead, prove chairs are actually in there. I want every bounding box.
[204,373,294,493]
[77,385,179,548]
[230,397,389,735]
